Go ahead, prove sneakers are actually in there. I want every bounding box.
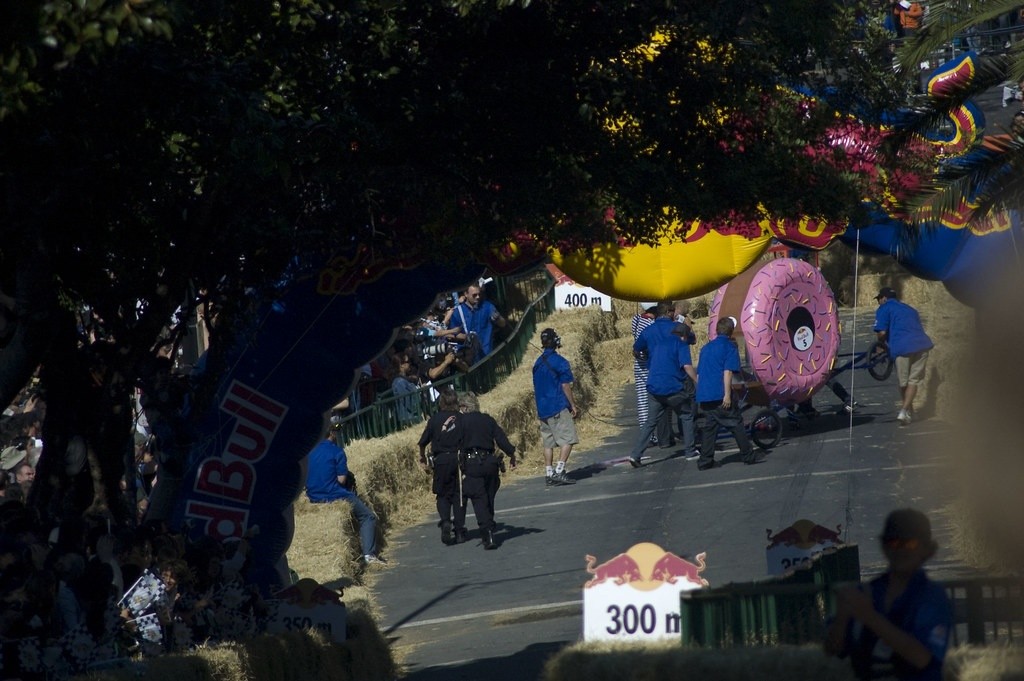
[364,554,386,566]
[545,475,560,486]
[551,469,576,484]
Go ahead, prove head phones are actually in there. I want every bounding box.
[541,333,561,345]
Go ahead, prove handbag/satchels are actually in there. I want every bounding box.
[457,334,479,367]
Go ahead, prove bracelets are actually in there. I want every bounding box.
[443,424,447,426]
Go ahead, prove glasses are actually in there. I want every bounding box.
[468,292,481,298]
[885,539,920,550]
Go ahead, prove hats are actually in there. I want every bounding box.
[0,447,27,470]
[873,287,896,299]
[878,509,931,541]
[671,324,694,337]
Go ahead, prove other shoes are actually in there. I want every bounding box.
[441,520,451,544]
[482,530,497,550]
[836,400,856,414]
[455,532,472,543]
[685,452,700,461]
[896,412,912,425]
[744,453,766,465]
[699,461,721,471]
[628,457,641,467]
[794,406,816,416]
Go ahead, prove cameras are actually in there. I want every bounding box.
[416,342,448,357]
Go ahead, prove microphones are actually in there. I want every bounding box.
[675,315,695,325]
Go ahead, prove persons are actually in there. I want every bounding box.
[695,318,756,471]
[793,376,862,417]
[356,282,506,438]
[305,424,384,564]
[416,390,469,544]
[823,509,953,681]
[0,387,250,681]
[532,328,578,487]
[628,301,699,468]
[871,287,934,424]
[440,392,517,550]
